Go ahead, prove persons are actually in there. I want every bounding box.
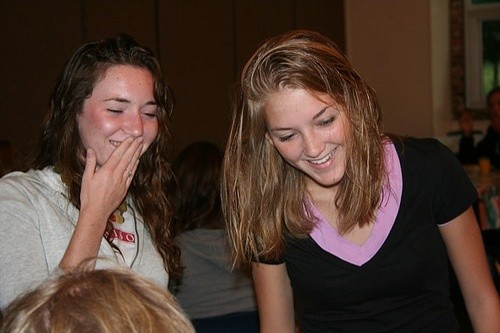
[220,29,500,333]
[0,34,261,333]
[459,87,500,170]
